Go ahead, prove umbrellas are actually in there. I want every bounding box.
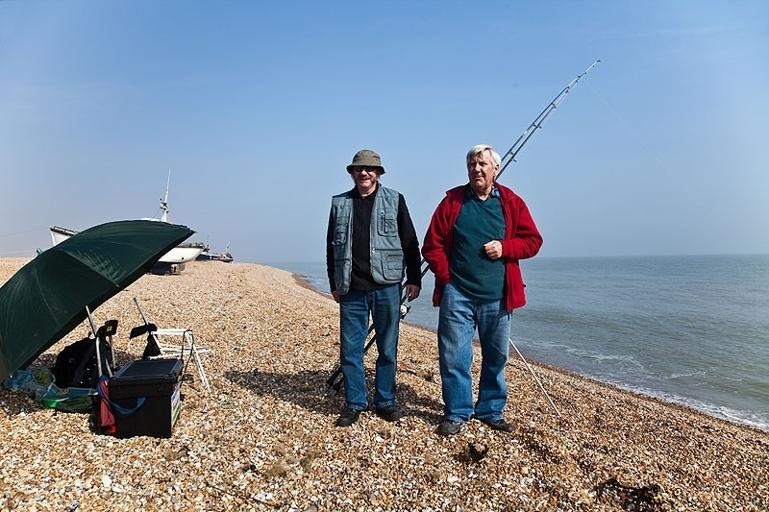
[0,218,198,390]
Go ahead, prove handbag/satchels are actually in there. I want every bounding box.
[54,337,110,390]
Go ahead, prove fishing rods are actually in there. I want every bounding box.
[327,60,601,393]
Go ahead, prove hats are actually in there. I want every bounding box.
[346,150,386,175]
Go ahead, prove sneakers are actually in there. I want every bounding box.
[380,405,399,422]
[439,419,463,436]
[337,408,359,427]
[488,418,513,432]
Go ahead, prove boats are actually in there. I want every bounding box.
[49,165,206,275]
[188,241,235,263]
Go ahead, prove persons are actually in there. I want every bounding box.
[325,149,424,427]
[419,143,543,435]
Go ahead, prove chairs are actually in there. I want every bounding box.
[130,295,213,393]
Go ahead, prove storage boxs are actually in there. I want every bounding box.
[107,359,181,437]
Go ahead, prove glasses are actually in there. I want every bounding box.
[354,165,375,173]
